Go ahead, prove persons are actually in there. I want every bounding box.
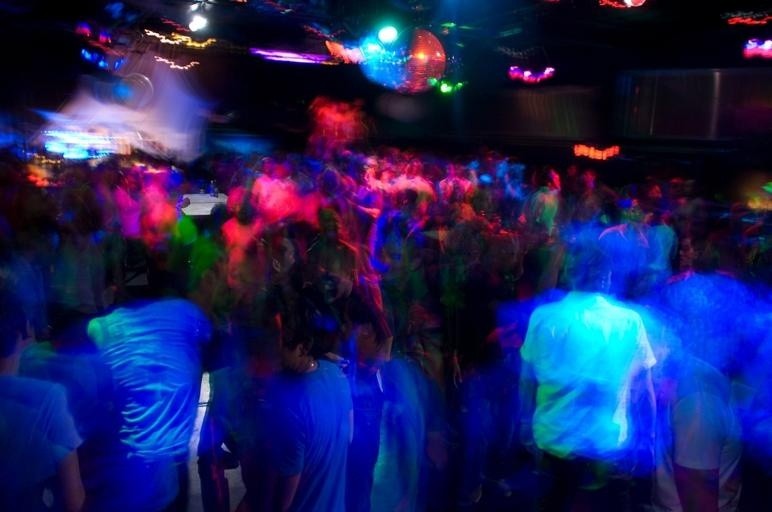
[2,95,772,512]
